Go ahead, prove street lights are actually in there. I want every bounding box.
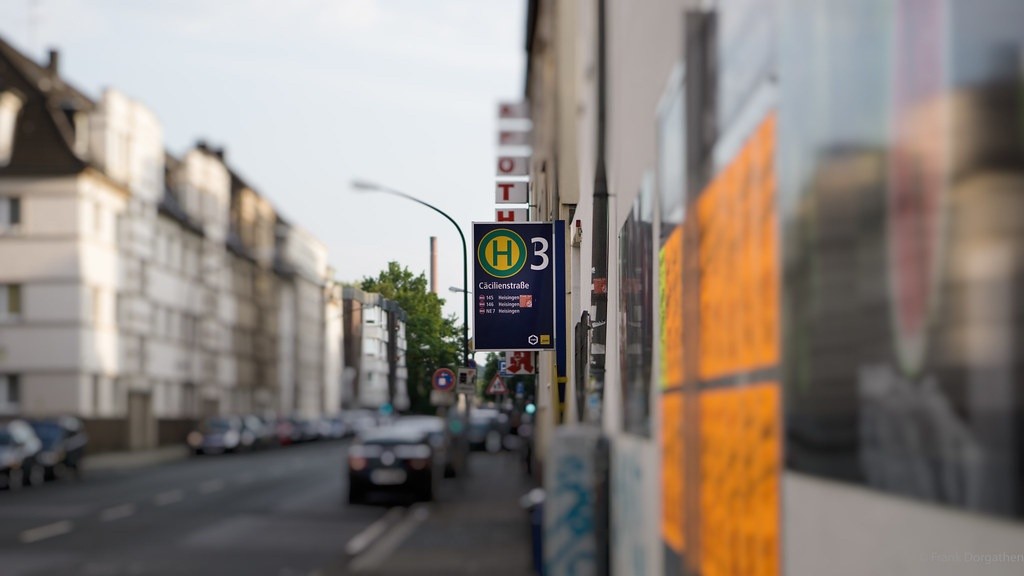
[344,180,472,413]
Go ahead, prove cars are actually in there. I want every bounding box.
[468,408,511,451]
[0,414,89,491]
[347,414,468,502]
[187,409,393,457]
